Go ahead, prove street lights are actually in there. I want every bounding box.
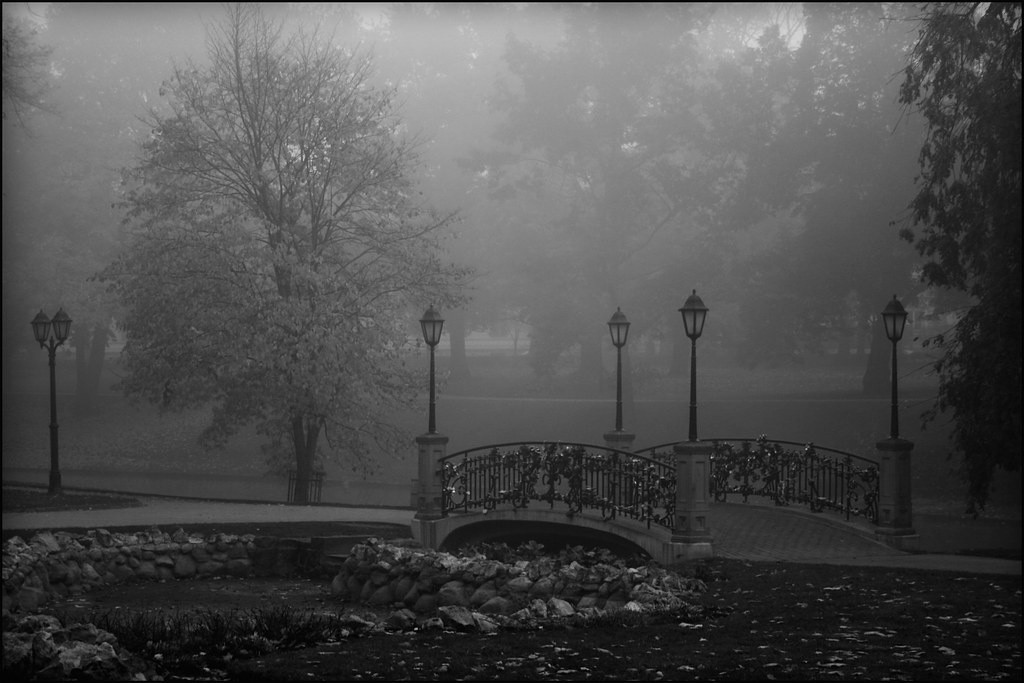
[28,304,73,494]
[417,302,447,436]
[879,292,909,439]
[676,287,710,442]
[605,304,632,431]
